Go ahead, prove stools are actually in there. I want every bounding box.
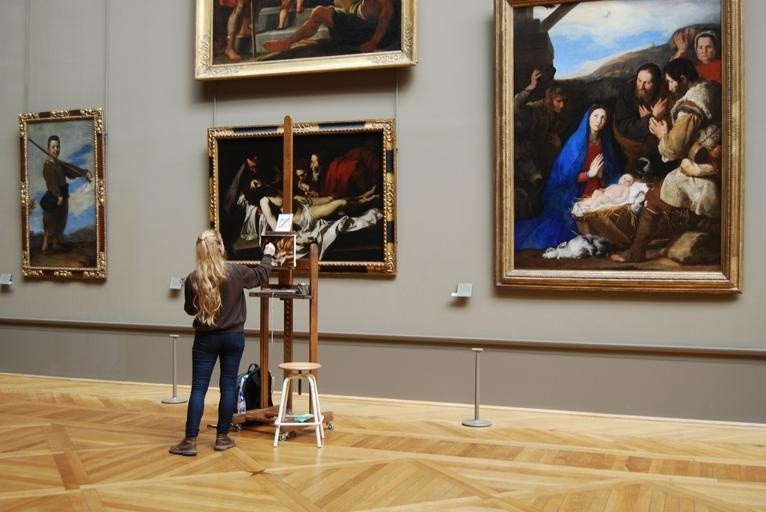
[272,361,325,448]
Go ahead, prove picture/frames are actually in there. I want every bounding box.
[205,116,397,280]
[490,0,744,296]
[18,106,106,282]
[196,0,419,81]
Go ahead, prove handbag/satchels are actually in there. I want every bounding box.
[234,365,272,428]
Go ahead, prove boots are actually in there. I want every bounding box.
[215,434,235,450]
[170,437,197,455]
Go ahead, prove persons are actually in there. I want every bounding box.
[40,135,92,251]
[278,0,304,29]
[219,140,381,264]
[169,230,276,455]
[217,0,261,62]
[261,1,396,51]
